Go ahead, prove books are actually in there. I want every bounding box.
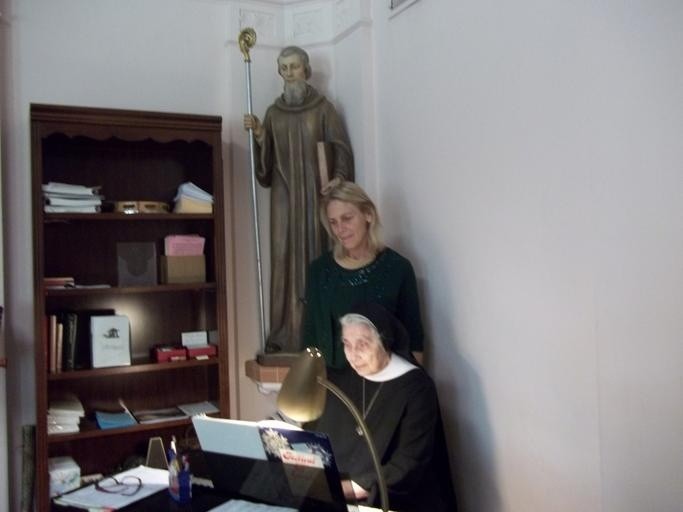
[50,311,131,375]
[192,412,348,512]
[116,242,157,287]
[42,182,105,214]
[46,393,85,435]
[45,276,75,290]
[132,402,189,424]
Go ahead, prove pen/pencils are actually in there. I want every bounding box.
[168,441,190,471]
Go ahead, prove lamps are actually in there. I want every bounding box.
[276,343,390,512]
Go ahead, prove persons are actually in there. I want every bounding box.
[244,47,354,355]
[302,181,423,373]
[302,302,458,512]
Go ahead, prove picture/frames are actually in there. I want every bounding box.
[384,0,417,20]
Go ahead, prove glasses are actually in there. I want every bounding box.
[94,474,142,496]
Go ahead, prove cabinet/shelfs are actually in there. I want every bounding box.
[29,100,231,510]
